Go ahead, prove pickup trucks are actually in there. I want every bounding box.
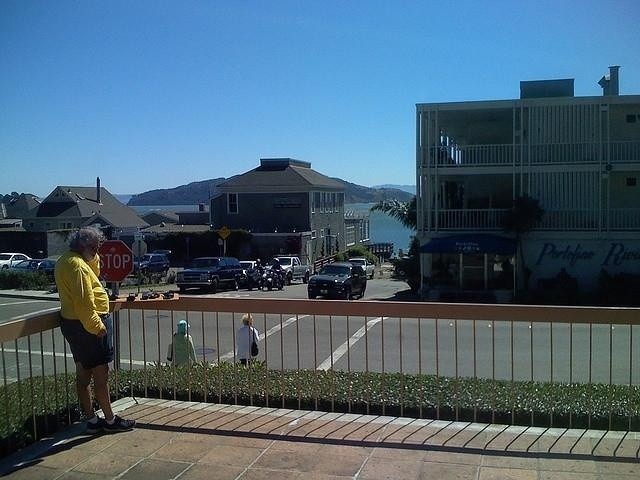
[263,257,311,286]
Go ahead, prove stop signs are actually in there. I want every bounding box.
[97,238,135,285]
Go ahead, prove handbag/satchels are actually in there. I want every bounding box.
[167,343,173,361]
[251,342,259,356]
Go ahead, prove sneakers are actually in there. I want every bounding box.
[104,416,135,432]
[85,414,105,434]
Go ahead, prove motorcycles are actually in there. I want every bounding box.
[244,266,263,291]
[264,269,286,291]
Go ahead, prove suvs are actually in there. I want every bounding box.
[239,260,258,286]
[307,262,367,301]
[348,258,375,279]
[174,257,246,294]
[139,253,170,276]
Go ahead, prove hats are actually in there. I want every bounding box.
[177,320,191,328]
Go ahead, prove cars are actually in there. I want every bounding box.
[13,259,57,281]
[0,252,33,270]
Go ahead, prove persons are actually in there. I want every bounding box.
[53,225,136,433]
[253,257,285,290]
[233,313,261,368]
[169,320,198,369]
